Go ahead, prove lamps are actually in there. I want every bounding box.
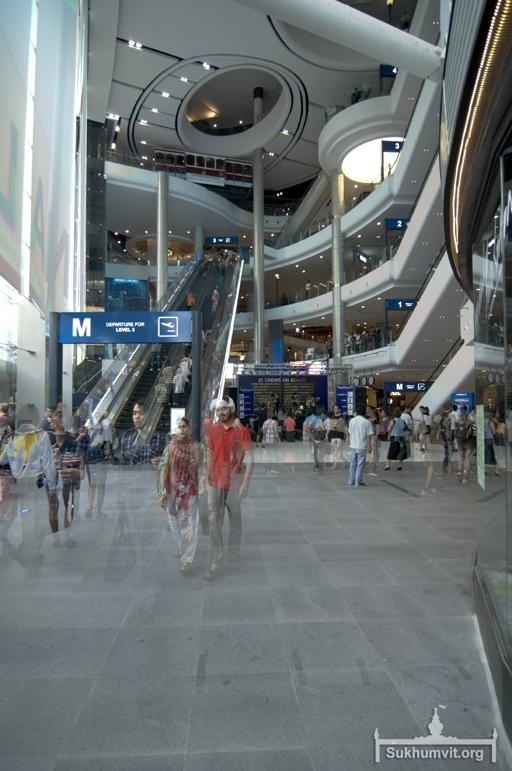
[316,96,412,188]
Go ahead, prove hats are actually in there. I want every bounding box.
[210,396,235,413]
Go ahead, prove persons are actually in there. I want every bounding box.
[0,249,506,580]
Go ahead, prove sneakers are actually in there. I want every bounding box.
[348,482,365,486]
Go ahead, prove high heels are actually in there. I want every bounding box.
[384,466,390,470]
[397,466,402,470]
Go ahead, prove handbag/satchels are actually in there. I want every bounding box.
[105,511,140,583]
[9,503,25,548]
